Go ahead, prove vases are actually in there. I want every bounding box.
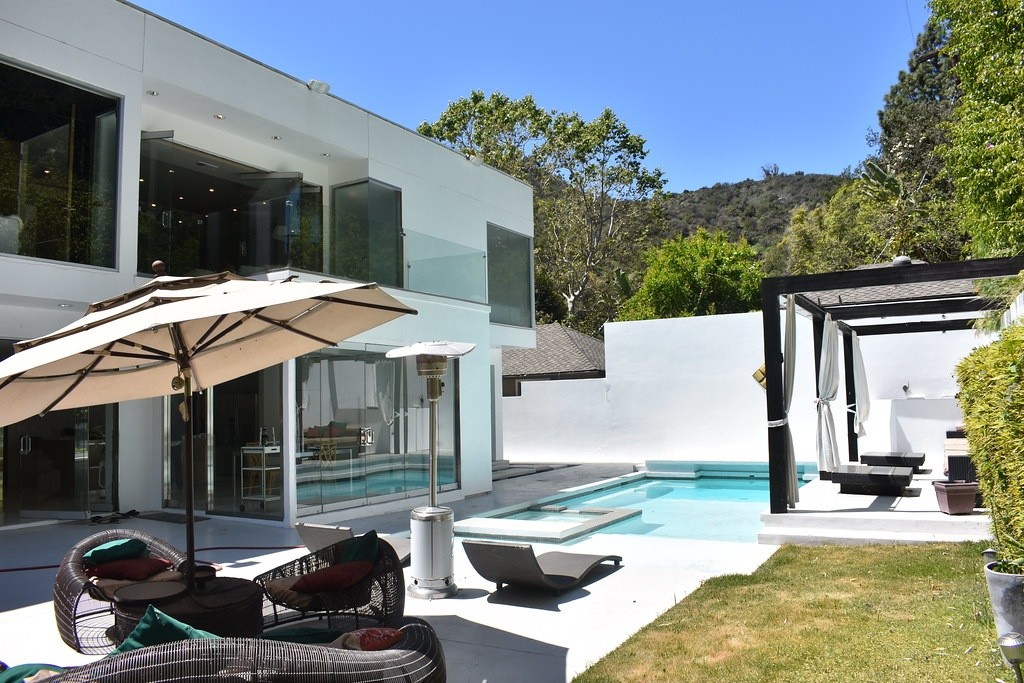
[933,479,979,515]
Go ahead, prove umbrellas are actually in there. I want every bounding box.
[0,270,419,594]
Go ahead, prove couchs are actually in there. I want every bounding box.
[304,427,362,459]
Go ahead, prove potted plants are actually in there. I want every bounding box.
[950,317,1024,662]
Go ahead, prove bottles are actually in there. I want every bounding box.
[263,426,269,447]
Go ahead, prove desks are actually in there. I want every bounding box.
[115,577,264,648]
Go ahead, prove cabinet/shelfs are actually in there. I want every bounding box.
[240,447,280,501]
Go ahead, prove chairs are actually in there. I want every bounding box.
[461,541,622,593]
[53,528,186,651]
[25,617,448,683]
[253,537,405,628]
[294,521,411,565]
[0,217,22,255]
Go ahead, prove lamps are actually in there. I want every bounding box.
[753,363,766,390]
[465,153,484,167]
[307,79,330,94]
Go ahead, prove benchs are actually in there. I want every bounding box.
[861,452,926,473]
[832,465,914,496]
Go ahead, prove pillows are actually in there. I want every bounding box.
[307,421,347,438]
[105,603,221,656]
[82,556,172,581]
[327,628,404,651]
[82,538,151,564]
[337,529,381,564]
[289,562,374,593]
